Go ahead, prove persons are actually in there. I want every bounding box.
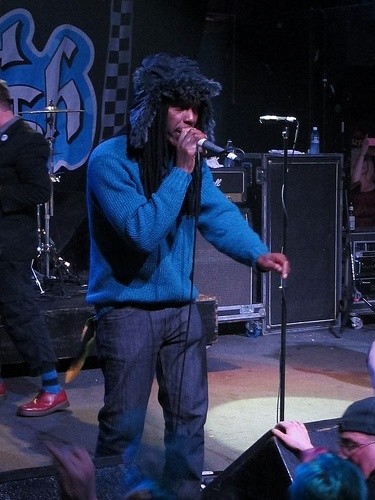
[0,78,70,417]
[41,439,176,500]
[286,451,368,500]
[271,395,375,500]
[86,52,290,500]
[348,130,375,227]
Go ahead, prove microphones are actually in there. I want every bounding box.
[196,138,241,163]
[259,115,297,122]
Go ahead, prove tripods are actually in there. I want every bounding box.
[36,112,80,295]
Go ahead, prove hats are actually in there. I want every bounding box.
[128,52,222,148]
[339,397,375,436]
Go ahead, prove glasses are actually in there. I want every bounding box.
[338,441,375,458]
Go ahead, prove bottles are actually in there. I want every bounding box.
[223,138,234,168]
[310,127,319,155]
[349,202,356,232]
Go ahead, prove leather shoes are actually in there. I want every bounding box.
[1,383,7,401]
[18,387,70,417]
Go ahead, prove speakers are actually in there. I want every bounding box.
[200,417,346,500]
[192,207,254,307]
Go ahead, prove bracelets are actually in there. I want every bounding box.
[360,152,366,157]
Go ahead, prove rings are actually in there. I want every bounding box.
[282,260,288,267]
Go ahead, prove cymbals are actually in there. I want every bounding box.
[16,106,86,115]
